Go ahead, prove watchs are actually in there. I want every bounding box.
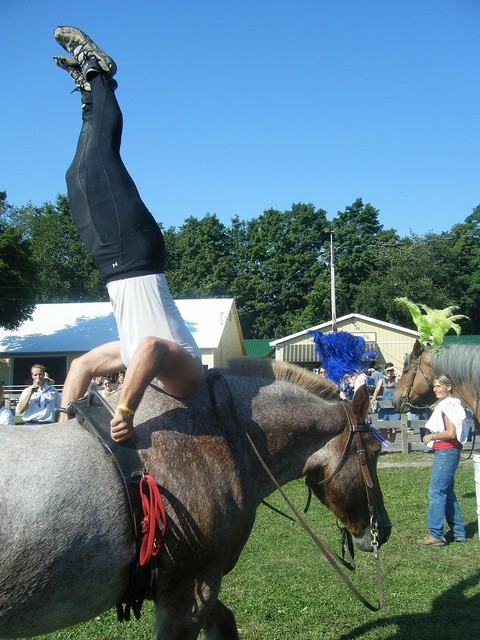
[430,434,435,441]
[116,403,135,418]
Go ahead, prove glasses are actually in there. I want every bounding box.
[104,381,111,386]
[320,370,327,374]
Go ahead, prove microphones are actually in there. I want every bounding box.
[33,378,40,393]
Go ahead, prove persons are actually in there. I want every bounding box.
[88,377,103,393]
[98,378,116,397]
[370,364,401,444]
[44,373,60,423]
[116,374,125,390]
[416,373,470,547]
[339,365,382,400]
[317,367,326,378]
[0,385,15,426]
[51,26,202,441]
[16,364,55,426]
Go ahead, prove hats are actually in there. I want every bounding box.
[383,367,396,373]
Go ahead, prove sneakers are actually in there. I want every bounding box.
[55,57,91,91]
[55,27,117,81]
[416,533,446,548]
[444,528,467,544]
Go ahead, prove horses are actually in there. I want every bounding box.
[392,339,480,425]
[1,358,393,640]
